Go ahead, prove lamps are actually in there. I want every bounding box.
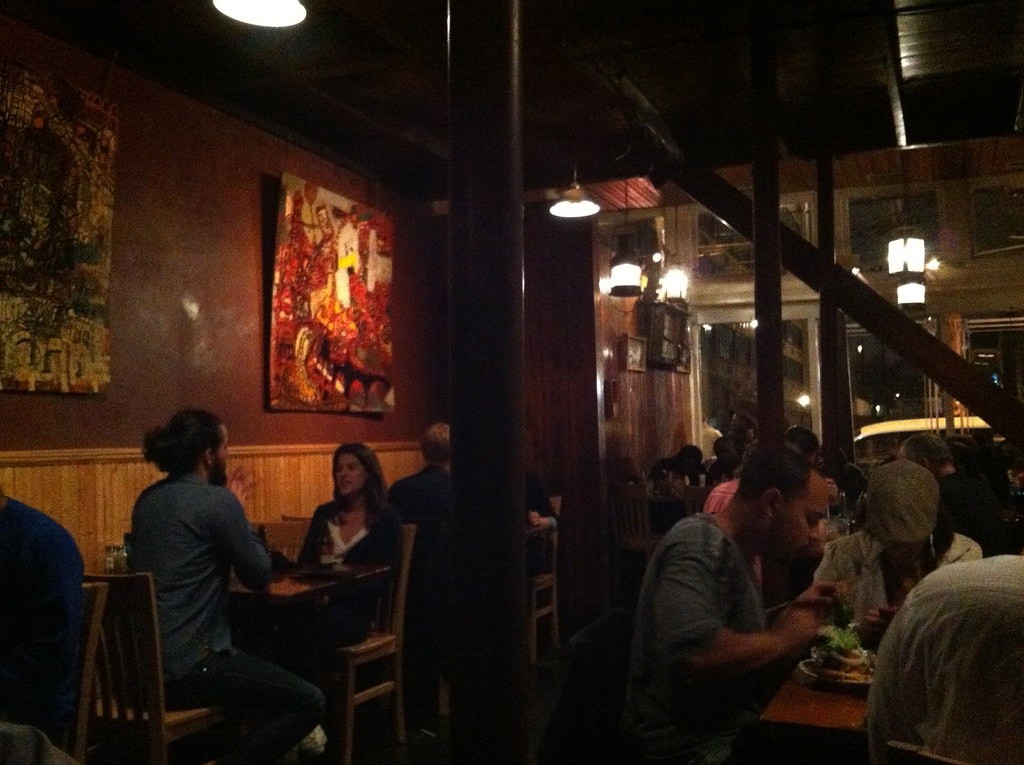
[664,207,688,302]
[550,166,600,219]
[896,280,928,312]
[610,178,642,297]
[888,154,925,283]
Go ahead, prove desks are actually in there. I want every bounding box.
[227,559,389,608]
[759,671,868,737]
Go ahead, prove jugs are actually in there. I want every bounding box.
[104,544,127,574]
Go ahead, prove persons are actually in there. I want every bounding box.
[953,429,1007,480]
[620,412,867,600]
[277,442,402,761]
[621,444,842,765]
[896,432,1007,555]
[124,409,327,765]
[0,488,85,740]
[388,420,453,519]
[518,464,559,578]
[867,543,1024,765]
[812,459,985,639]
[729,414,758,442]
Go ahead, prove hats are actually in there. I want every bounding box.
[863,460,940,544]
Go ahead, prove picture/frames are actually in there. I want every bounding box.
[690,173,1024,305]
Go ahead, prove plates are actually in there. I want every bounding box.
[796,658,875,687]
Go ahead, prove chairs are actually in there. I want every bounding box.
[72,481,723,764]
